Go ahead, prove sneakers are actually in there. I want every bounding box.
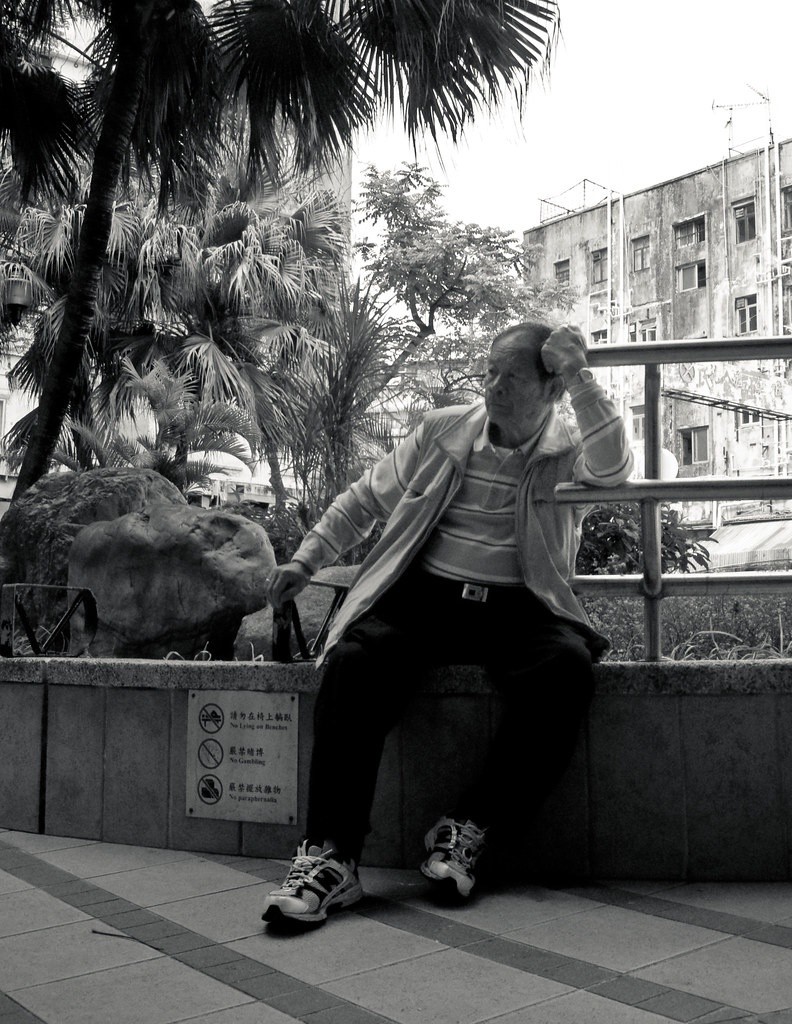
[419,816,491,901]
[262,833,365,926]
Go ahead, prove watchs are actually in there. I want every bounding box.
[566,368,593,389]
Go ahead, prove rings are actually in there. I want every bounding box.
[265,578,270,582]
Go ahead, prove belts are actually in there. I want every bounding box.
[409,567,533,607]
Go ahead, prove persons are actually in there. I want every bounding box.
[261,322,632,927]
